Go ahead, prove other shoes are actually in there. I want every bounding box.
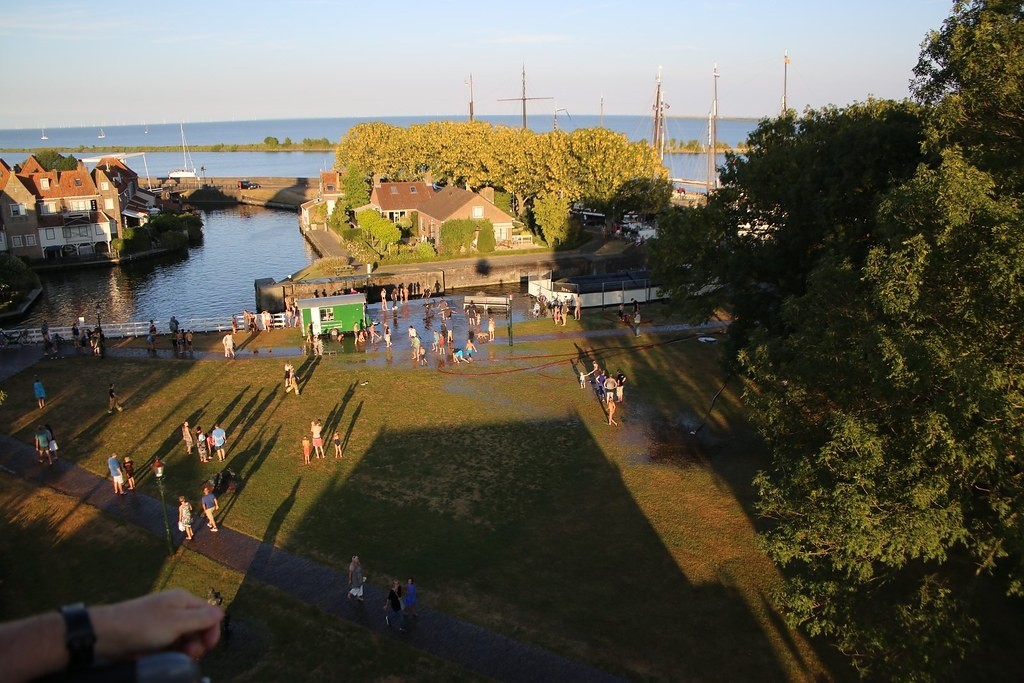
[400,627,407,632]
[37,459,43,463]
[207,523,213,528]
[386,616,391,625]
[358,597,363,600]
[186,536,192,539]
[211,527,218,532]
[49,462,53,466]
[203,460,208,463]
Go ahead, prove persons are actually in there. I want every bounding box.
[107,383,123,413]
[452,293,582,364]
[178,496,194,539]
[34,424,58,466]
[33,379,46,409]
[108,452,136,495]
[284,286,458,365]
[146,316,194,354]
[222,309,272,358]
[302,419,343,464]
[383,578,419,632]
[181,421,227,463]
[202,487,219,531]
[346,555,367,602]
[284,360,301,395]
[41,320,106,360]
[618,297,641,338]
[578,360,627,426]
[0,588,225,683]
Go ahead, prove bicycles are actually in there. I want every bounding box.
[0,325,32,350]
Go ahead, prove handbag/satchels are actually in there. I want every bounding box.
[399,599,406,610]
[49,440,58,452]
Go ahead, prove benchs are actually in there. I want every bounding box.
[1,331,32,348]
[334,265,355,276]
[463,296,511,310]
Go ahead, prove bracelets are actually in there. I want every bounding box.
[59,602,97,669]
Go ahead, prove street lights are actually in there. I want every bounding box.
[94,302,103,339]
[150,454,176,556]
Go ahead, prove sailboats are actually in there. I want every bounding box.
[40,127,48,140]
[628,66,718,210]
[168,120,198,177]
[98,129,106,138]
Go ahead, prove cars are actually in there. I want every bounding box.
[238,180,257,188]
